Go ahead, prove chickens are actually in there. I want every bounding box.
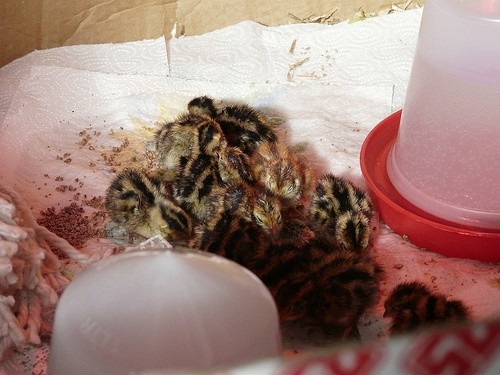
[105,96,470,340]
